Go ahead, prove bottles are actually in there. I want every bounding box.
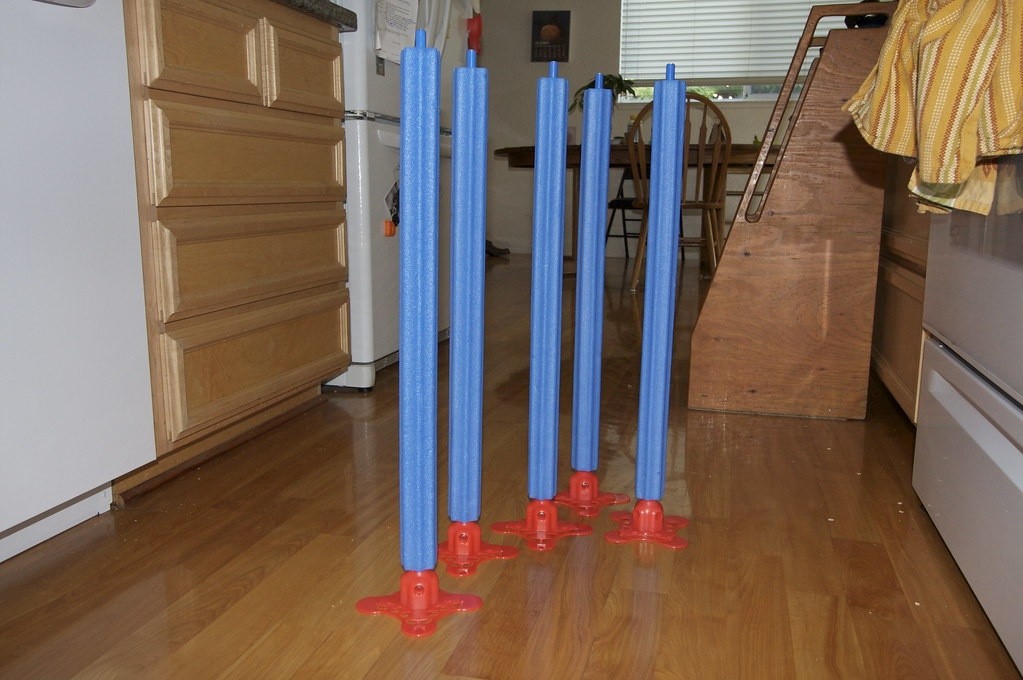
[627,115,644,143]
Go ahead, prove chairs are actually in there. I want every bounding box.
[602,163,685,262]
[626,91,733,292]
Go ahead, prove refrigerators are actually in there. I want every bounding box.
[327,1,463,389]
[913,157,1023,680]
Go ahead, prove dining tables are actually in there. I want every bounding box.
[493,142,782,275]
